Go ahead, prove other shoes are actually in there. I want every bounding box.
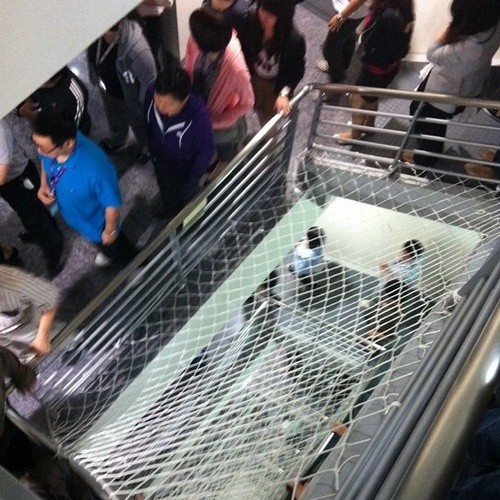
[313,90,327,101]
[346,93,352,105]
[20,229,34,244]
[207,160,219,173]
[153,203,171,218]
[6,246,19,267]
[402,151,415,163]
[98,141,127,155]
[317,59,348,76]
[136,140,150,164]
[333,133,352,145]
[465,147,497,184]
[47,260,59,281]
[347,120,375,135]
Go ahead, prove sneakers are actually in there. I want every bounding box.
[483,108,500,123]
[94,251,111,267]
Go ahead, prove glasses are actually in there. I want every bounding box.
[31,138,57,155]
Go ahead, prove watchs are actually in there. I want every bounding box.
[337,11,347,23]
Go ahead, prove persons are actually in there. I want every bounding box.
[0,1,499,499]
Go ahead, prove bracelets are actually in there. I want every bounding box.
[279,91,289,96]
